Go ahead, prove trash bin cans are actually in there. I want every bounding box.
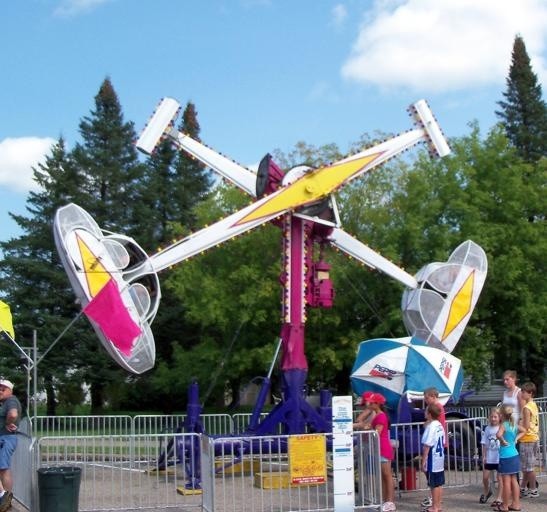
[36,467,82,512]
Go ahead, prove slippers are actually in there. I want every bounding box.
[480,492,521,511]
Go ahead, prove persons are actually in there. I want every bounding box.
[352,371,540,512]
[0,379,23,512]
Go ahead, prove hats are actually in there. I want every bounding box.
[360,390,386,405]
[1,379,16,391]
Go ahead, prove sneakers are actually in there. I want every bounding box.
[0,489,13,512]
[420,496,443,512]
[520,486,539,499]
[380,501,397,511]
